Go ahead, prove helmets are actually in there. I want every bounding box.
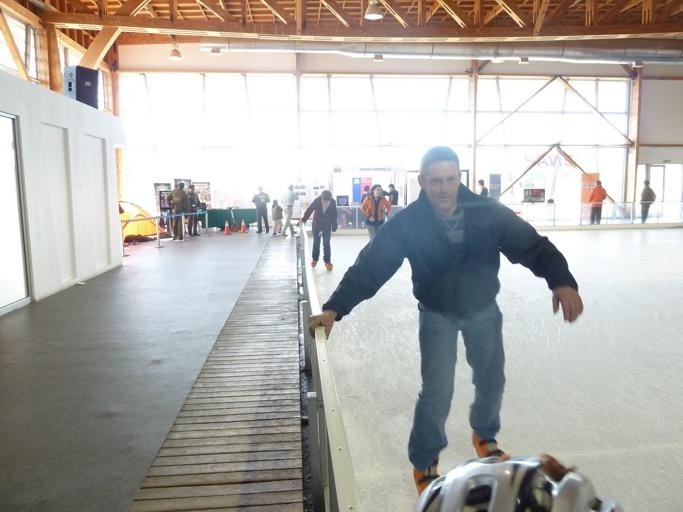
[414,457,624,512]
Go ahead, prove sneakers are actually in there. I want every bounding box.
[325,262,332,270]
[414,463,440,495]
[473,431,510,462]
[311,260,316,268]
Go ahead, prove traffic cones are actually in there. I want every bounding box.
[223,219,232,235]
[240,218,247,233]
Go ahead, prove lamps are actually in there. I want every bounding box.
[169,42,181,60]
[364,0,382,19]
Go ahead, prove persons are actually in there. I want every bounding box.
[588,181,605,225]
[167,183,200,240]
[300,190,337,270]
[252,184,299,236]
[360,184,399,239]
[308,146,583,496]
[479,179,488,197]
[640,180,657,223]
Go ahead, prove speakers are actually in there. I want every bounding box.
[63,66,98,110]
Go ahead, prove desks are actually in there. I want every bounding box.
[200,208,257,230]
